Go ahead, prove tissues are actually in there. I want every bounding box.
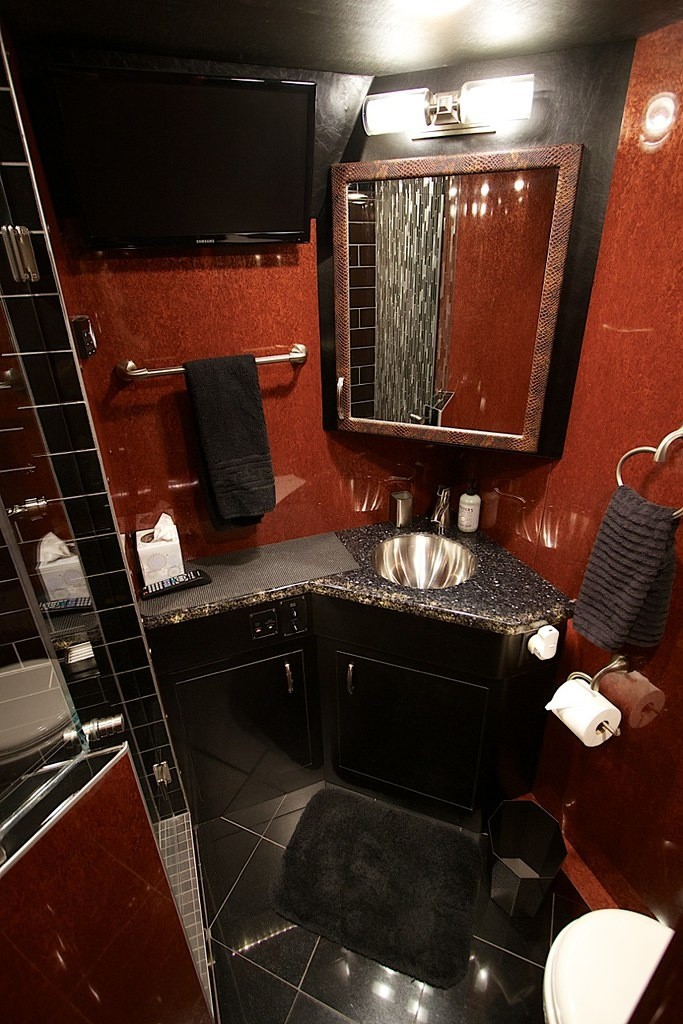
[35,531,93,601]
[135,511,185,586]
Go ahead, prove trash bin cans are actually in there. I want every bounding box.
[489,798,569,918]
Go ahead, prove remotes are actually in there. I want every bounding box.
[37,597,93,612]
[141,569,212,600]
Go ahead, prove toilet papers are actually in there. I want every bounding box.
[599,669,666,729]
[544,678,622,747]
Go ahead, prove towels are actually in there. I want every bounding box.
[571,484,679,655]
[181,352,277,519]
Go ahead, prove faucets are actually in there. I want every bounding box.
[430,485,452,527]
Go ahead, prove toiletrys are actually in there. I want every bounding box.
[458,478,482,533]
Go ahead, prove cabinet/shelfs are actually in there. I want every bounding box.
[144,594,567,835]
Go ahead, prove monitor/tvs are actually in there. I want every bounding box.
[50,65,316,250]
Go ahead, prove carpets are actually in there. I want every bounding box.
[266,787,483,989]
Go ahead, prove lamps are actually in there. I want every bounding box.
[362,73,534,140]
[639,93,679,152]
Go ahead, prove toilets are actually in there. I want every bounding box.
[539,907,675,1024]
[0,657,114,769]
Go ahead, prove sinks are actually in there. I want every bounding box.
[367,531,478,590]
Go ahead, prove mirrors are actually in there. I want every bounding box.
[348,167,563,436]
